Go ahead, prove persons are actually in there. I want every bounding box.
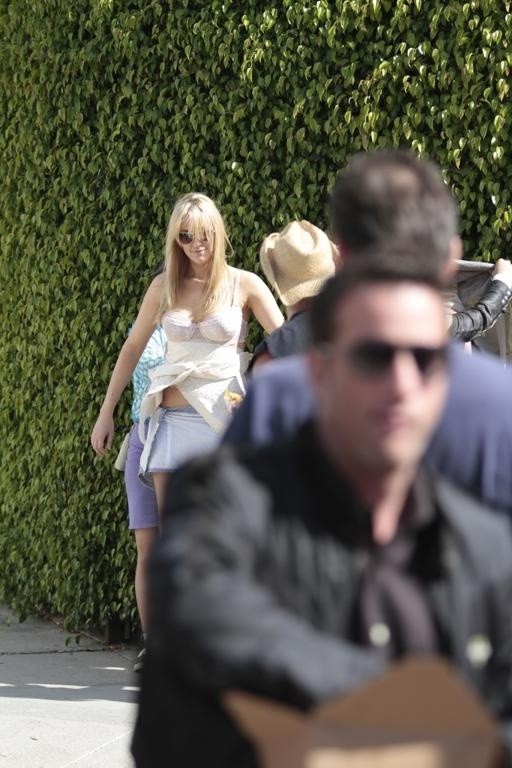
[220,147,512,528]
[241,215,512,388]
[205,648,512,768]
[125,249,511,768]
[113,284,167,674]
[89,190,292,540]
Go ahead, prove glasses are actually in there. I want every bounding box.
[177,231,208,245]
[341,343,447,383]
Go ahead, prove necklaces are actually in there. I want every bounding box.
[192,276,206,284]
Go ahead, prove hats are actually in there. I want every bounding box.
[258,218,342,306]
